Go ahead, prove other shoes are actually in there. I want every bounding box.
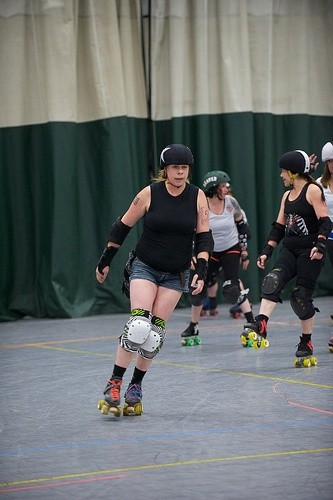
[328,337,333,353]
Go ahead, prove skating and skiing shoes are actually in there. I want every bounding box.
[229,301,252,319]
[240,320,256,347]
[98,377,122,417]
[242,314,269,349]
[295,336,318,368]
[180,323,202,346]
[122,383,143,415]
[200,298,218,316]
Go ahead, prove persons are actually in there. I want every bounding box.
[308,142,333,353]
[243,148,333,368]
[181,171,255,347]
[95,145,214,416]
[200,185,252,319]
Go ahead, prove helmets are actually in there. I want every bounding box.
[159,143,195,170]
[201,170,230,191]
[278,150,310,174]
[321,141,333,162]
